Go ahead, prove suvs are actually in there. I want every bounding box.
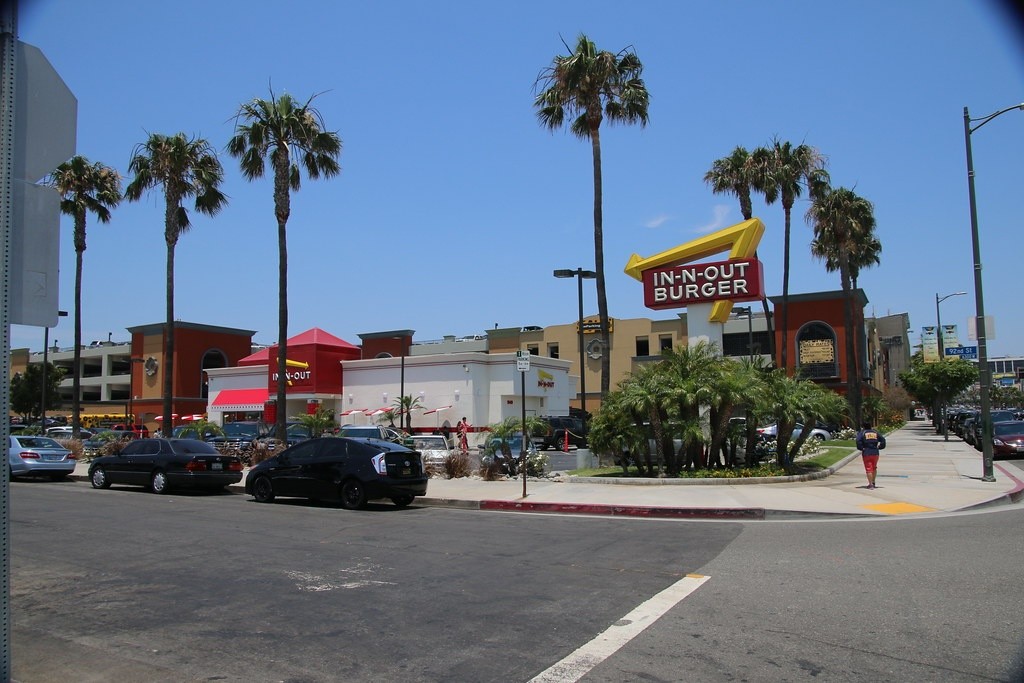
[337,423,392,444]
[611,421,705,468]
[112,424,149,439]
[532,414,593,452]
[384,427,412,446]
[206,421,270,464]
[261,421,318,461]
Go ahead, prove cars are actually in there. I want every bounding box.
[173,425,206,442]
[730,417,748,436]
[245,435,429,510]
[992,421,1024,460]
[755,420,831,446]
[928,405,1024,446]
[974,410,1018,452]
[8,435,77,481]
[407,435,453,472]
[86,438,244,494]
[10,418,123,453]
[476,431,537,475]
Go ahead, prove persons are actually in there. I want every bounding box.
[856,421,886,489]
[433,428,442,435]
[442,426,449,440]
[456,421,465,450]
[460,416,473,449]
[153,430,161,438]
[157,428,162,438]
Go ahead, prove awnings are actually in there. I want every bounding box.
[211,388,269,412]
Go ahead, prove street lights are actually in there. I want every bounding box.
[963,103,1024,483]
[552,267,597,447]
[935,291,968,368]
[392,335,405,429]
[120,356,146,431]
[42,311,69,435]
[730,305,754,368]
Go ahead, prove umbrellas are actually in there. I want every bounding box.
[155,413,179,421]
[181,414,204,425]
[339,405,453,431]
